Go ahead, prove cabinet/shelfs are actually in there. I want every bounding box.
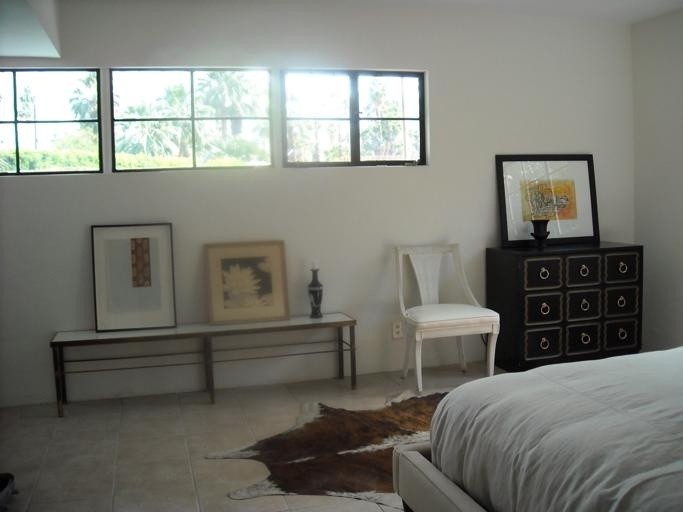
[484,242,647,374]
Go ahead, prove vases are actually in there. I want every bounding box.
[308,265,324,318]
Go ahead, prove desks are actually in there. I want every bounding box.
[50,313,356,416]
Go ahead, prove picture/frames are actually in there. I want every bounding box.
[89,223,287,334]
[493,152,599,247]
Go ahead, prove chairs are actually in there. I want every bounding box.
[394,240,500,392]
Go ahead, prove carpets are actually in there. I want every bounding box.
[204,389,445,509]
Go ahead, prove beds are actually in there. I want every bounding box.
[392,348,683,511]
